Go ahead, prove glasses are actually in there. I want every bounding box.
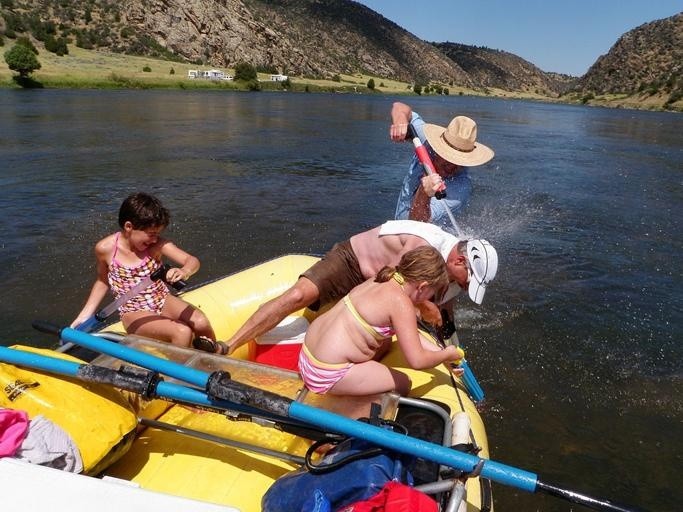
[467,268,472,283]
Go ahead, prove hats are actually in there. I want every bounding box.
[423,116,495,167]
[466,239,498,305]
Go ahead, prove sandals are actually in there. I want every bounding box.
[192,335,228,356]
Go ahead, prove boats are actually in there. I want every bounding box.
[0,251,494,512]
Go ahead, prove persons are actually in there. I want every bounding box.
[193,220,499,356]
[69,192,216,363]
[388,101,495,228]
[298,245,465,452]
